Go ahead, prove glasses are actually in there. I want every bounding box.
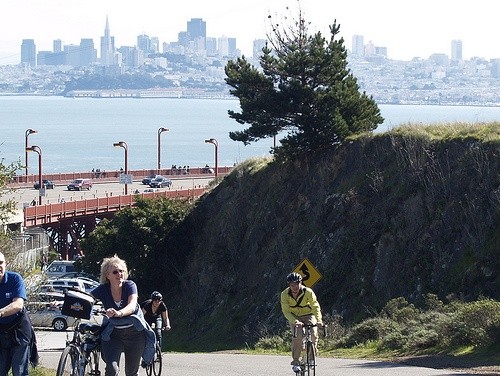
[110,270,124,275]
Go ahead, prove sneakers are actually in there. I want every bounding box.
[308,360,314,370]
[292,365,301,371]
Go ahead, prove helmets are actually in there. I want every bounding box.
[286,272,302,283]
[151,291,162,299]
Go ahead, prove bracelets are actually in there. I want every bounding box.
[119,310,123,318]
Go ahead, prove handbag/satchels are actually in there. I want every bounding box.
[59,288,96,320]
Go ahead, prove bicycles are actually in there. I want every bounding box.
[45,291,110,376]
[143,328,164,376]
[293,323,327,376]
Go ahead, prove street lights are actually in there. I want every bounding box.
[26,145,42,204]
[113,141,128,195]
[157,127,169,174]
[205,138,218,184]
[25,128,39,182]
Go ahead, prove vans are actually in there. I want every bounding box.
[67,179,92,191]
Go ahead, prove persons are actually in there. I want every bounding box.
[92,168,123,179]
[0,252,34,376]
[281,273,323,372]
[171,164,209,174]
[140,292,171,368]
[39,253,47,271]
[51,255,146,376]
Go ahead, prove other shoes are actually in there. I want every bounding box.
[141,361,147,369]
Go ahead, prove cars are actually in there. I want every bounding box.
[26,260,104,331]
[149,177,172,188]
[141,175,160,185]
[34,180,55,189]
